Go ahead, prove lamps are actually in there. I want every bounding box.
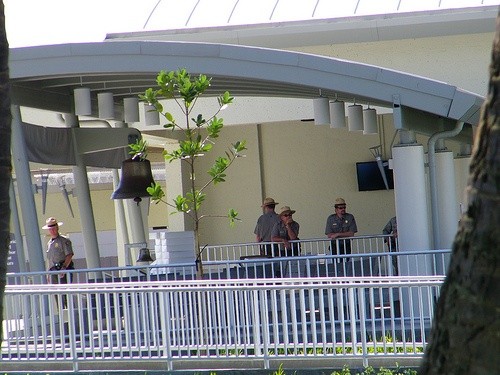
[313,88,330,126]
[348,98,363,132]
[329,93,346,128]
[97,82,115,120]
[363,102,378,135]
[74,77,92,115]
[123,89,140,123]
[144,100,160,125]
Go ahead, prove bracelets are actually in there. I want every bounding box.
[62,265,66,269]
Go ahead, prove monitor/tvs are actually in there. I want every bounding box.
[356,161,394,191]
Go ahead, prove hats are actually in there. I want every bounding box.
[261,198,279,207]
[334,198,346,205]
[278,206,296,217]
[42,217,63,229]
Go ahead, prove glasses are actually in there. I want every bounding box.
[282,214,292,217]
[337,207,346,209]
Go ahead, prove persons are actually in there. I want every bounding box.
[382,216,398,276]
[271,206,301,257]
[254,198,279,255]
[325,198,358,264]
[42,217,75,343]
